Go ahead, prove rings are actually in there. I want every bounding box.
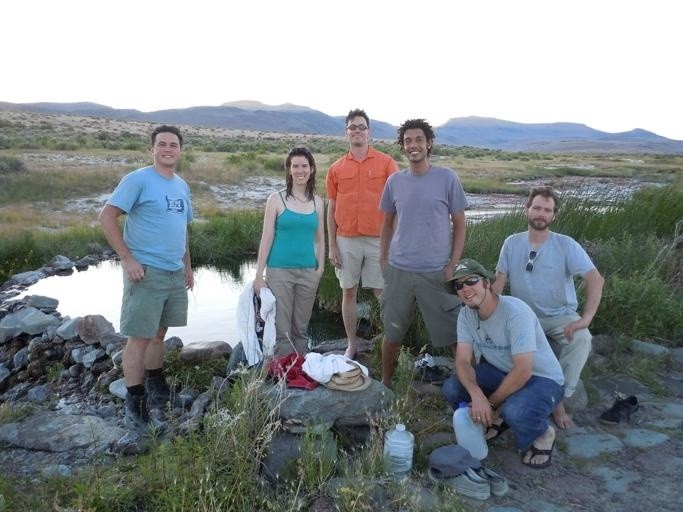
[476,417,480,418]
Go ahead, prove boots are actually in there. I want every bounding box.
[145,374,193,409]
[125,389,148,428]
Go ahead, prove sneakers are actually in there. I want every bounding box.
[427,467,491,500]
[598,395,640,425]
[412,363,430,383]
[475,465,508,497]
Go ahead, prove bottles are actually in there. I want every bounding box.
[382,424,415,488]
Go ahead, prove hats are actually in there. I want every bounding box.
[442,257,496,296]
[323,361,371,392]
[429,445,481,480]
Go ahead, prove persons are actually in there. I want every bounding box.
[326,109,399,360]
[379,119,470,391]
[443,258,565,468]
[489,187,604,430]
[98,125,195,427]
[252,147,325,358]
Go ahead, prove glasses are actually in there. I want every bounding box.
[525,251,538,272]
[452,276,485,290]
[348,124,367,130]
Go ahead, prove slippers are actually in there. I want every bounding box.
[431,363,451,385]
[522,428,558,468]
[485,417,509,444]
